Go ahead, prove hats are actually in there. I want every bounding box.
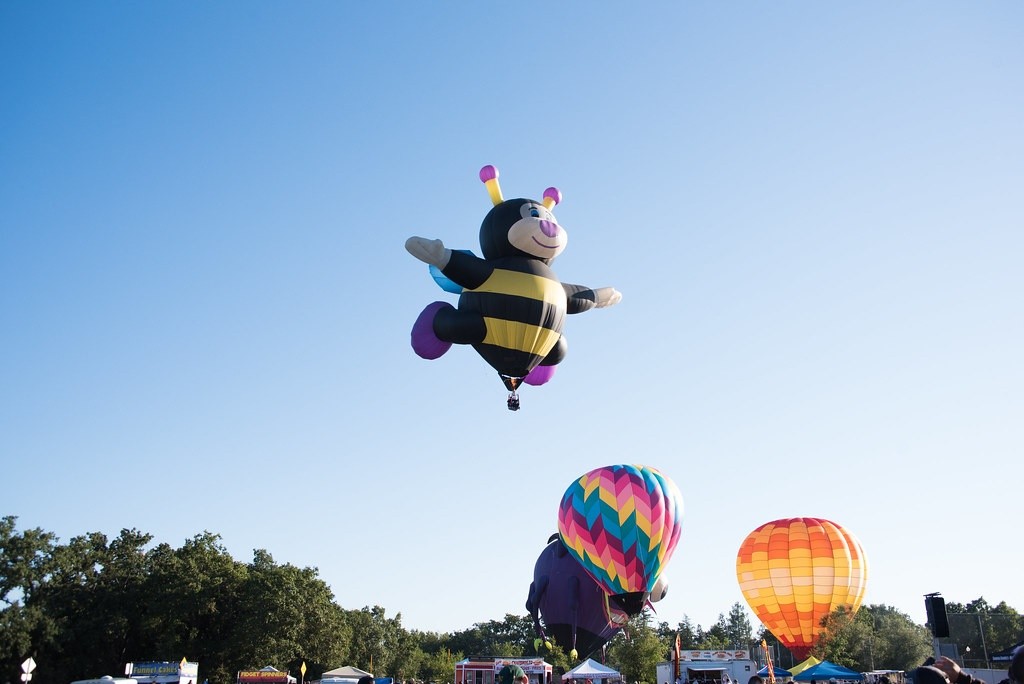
[902,666,950,684]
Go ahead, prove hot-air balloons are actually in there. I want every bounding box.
[403,164,623,411]
[736,517,869,663]
[557,465,684,636]
[525,532,669,662]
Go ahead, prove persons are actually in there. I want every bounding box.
[358,676,375,684]
[393,677,416,684]
[664,676,738,684]
[464,680,467,684]
[459,681,462,684]
[786,677,839,684]
[499,664,528,684]
[747,676,763,684]
[872,646,1024,684]
[559,677,626,684]
[304,680,311,684]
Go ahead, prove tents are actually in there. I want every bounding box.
[757,665,792,676]
[794,660,865,679]
[322,665,373,678]
[787,656,822,676]
[561,658,620,678]
[240,666,297,683]
